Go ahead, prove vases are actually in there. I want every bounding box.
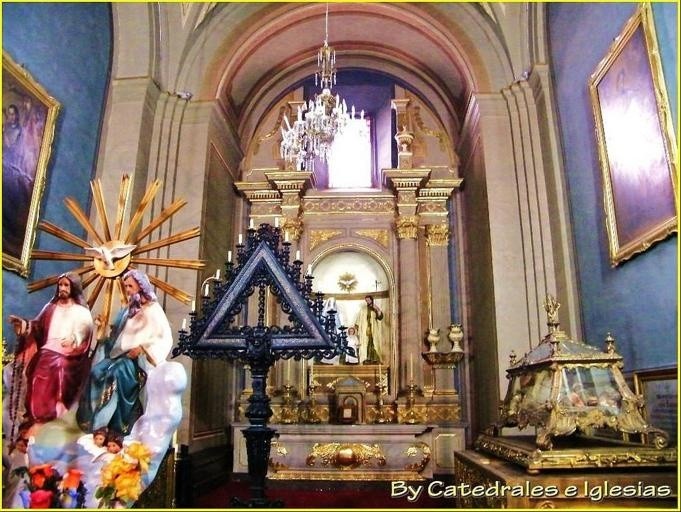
[427,327,441,351]
[447,324,465,352]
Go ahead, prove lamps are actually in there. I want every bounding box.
[276,4,370,168]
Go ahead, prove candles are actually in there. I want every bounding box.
[180,216,347,333]
[285,350,415,386]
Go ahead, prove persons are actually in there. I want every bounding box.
[3,101,32,227]
[8,268,171,509]
[322,294,385,365]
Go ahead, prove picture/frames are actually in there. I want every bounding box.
[3,50,62,279]
[586,3,678,270]
